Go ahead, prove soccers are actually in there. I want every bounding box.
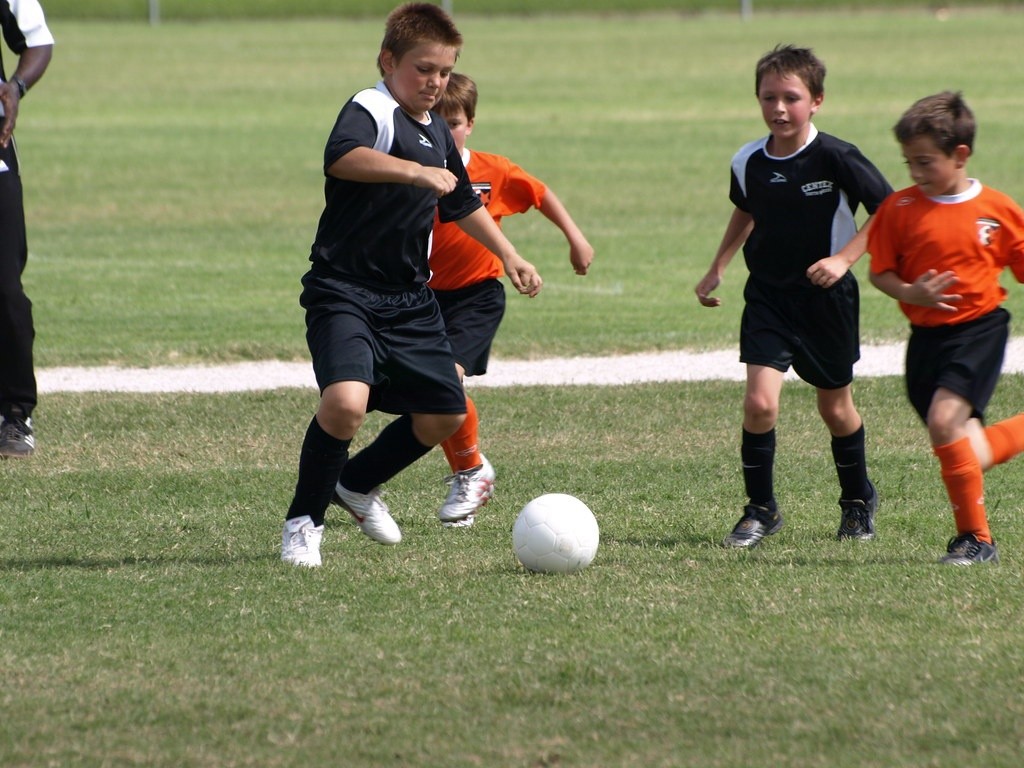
[510,492,600,575]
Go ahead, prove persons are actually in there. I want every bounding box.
[0,0,58,457]
[277,0,541,577]
[695,43,897,544]
[866,91,1024,568]
[426,72,593,528]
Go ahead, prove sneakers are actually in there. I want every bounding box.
[721,503,782,555]
[837,480,880,546]
[438,453,496,530]
[0,412,36,459]
[335,478,402,544]
[937,536,1000,572]
[280,513,323,567]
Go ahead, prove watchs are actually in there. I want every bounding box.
[12,76,26,98]
